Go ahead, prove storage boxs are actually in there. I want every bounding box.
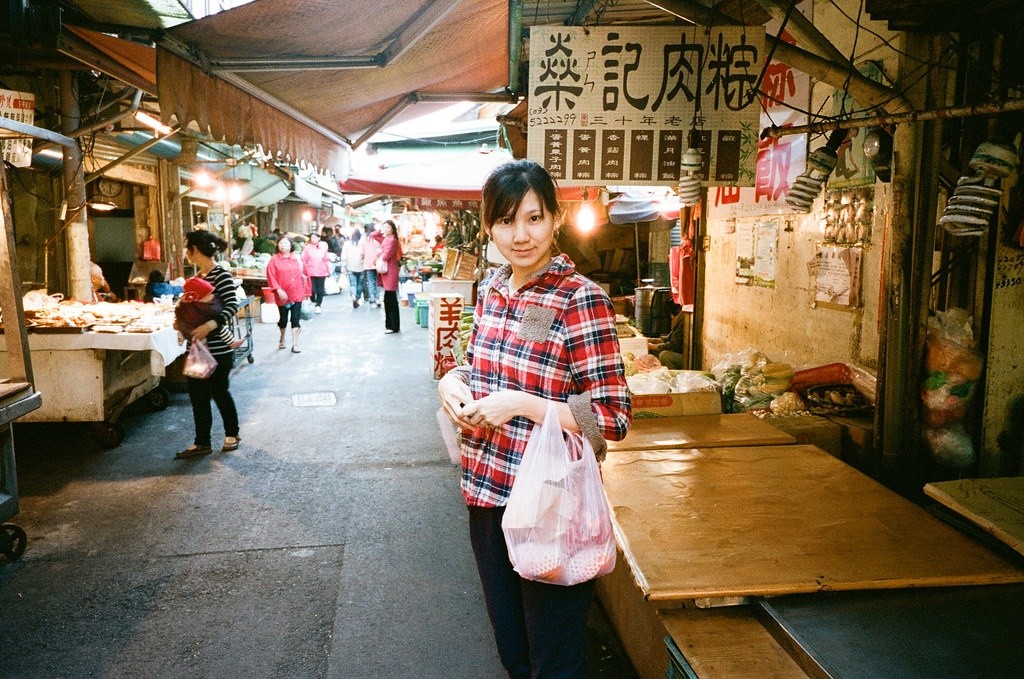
[428,279,475,305]
[626,373,721,419]
[442,247,462,280]
[261,287,275,304]
[764,416,842,459]
[260,302,291,324]
[454,247,479,281]
[413,298,428,324]
[398,282,422,300]
[238,295,261,319]
[829,414,873,449]
[418,302,429,328]
[407,292,415,308]
[400,275,428,283]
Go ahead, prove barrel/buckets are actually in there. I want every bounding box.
[262,287,276,303]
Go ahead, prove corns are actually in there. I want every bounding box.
[758,363,795,394]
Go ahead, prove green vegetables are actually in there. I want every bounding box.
[720,365,773,413]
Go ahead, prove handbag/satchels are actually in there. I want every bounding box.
[501,402,617,586]
[375,254,388,273]
[324,275,340,294]
[339,274,350,289]
[182,338,219,379]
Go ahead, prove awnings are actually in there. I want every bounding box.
[156,0,510,181]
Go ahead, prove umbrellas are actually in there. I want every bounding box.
[339,144,685,287]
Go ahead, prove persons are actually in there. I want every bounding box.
[300,223,384,314]
[430,235,444,257]
[370,220,400,334]
[90,260,187,305]
[266,236,313,353]
[173,230,247,459]
[437,158,633,679]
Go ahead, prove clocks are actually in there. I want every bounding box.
[98,178,123,198]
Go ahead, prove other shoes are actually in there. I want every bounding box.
[279,340,286,349]
[292,346,301,353]
[370,299,381,308]
[353,298,358,308]
[222,435,241,450]
[384,328,394,334]
[312,302,317,311]
[176,444,213,458]
[316,306,322,314]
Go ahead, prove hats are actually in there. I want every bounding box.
[183,276,216,303]
